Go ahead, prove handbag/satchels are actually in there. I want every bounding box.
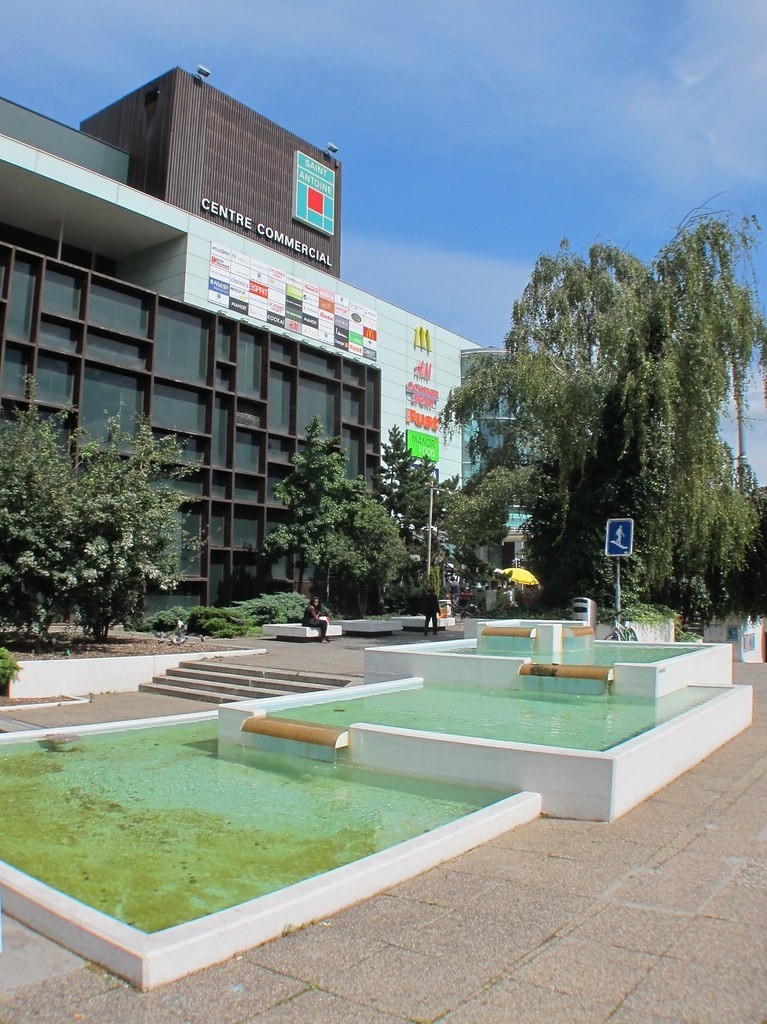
[318,616,330,626]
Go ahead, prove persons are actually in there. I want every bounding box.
[302,597,327,643]
[449,577,460,604]
[423,586,441,636]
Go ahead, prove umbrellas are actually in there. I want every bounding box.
[502,568,539,589]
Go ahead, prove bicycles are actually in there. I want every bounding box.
[441,593,480,620]
[605,608,638,641]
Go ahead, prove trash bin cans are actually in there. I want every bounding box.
[571,596,597,630]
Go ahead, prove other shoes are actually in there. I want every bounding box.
[321,639,328,643]
[424,628,428,636]
